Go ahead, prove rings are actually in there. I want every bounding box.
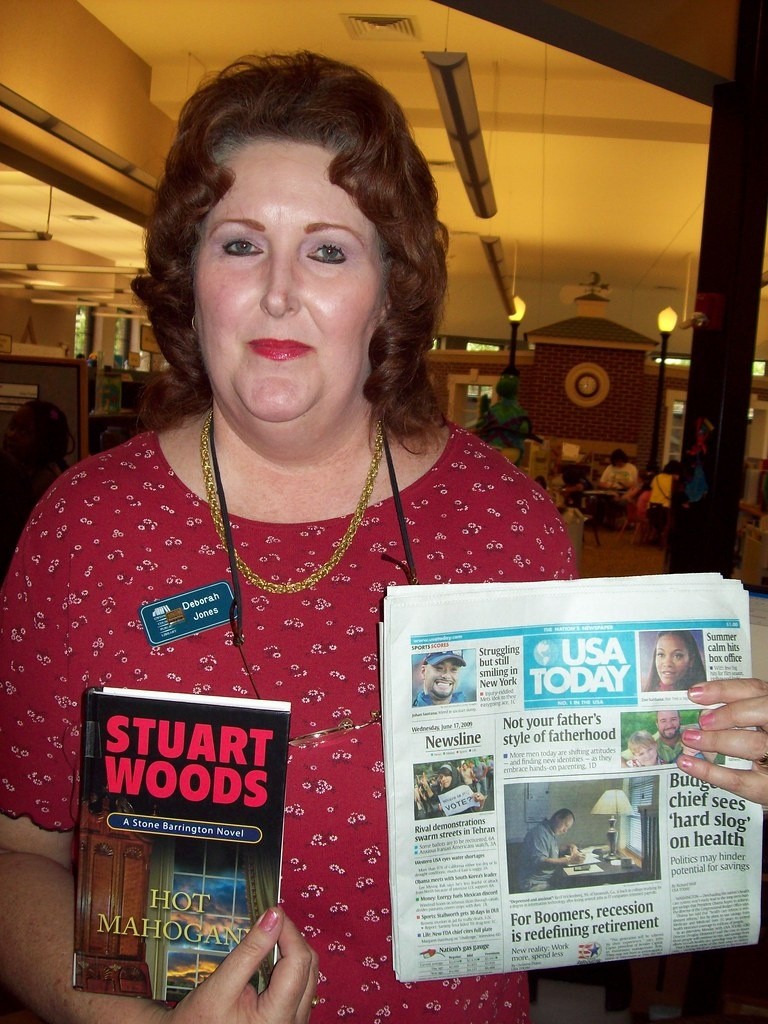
[755,752,768,768]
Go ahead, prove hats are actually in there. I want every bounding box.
[422,651,466,667]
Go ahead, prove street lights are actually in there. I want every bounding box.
[507,294,526,371]
[646,306,680,471]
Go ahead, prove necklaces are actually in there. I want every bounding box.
[199,408,386,591]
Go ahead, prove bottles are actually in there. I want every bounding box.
[85,351,125,414]
[100,426,126,451]
[732,456,768,568]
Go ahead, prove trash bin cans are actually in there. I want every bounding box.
[742,524,768,587]
[559,507,588,569]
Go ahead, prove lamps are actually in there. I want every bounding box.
[0,259,147,319]
[479,214,519,314]
[422,8,498,219]
[0,187,54,241]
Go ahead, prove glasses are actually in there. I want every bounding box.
[228,552,415,746]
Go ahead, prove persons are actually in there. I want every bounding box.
[414,757,493,821]
[0,48,768,1024]
[518,806,585,891]
[594,449,639,530]
[621,709,725,770]
[0,399,77,586]
[647,459,681,545]
[645,630,707,692]
[413,651,467,707]
[538,447,595,557]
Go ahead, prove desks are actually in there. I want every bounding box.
[581,490,626,545]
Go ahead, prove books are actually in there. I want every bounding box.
[71,685,292,1005]
